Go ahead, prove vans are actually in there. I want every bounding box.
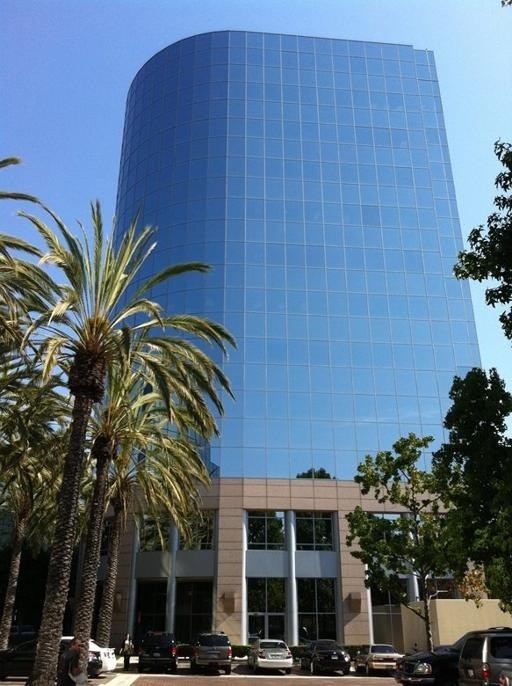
[456,631,511,686]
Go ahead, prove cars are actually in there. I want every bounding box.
[0,639,102,682]
[299,638,351,675]
[395,627,512,686]
[353,643,405,676]
[247,639,294,675]
[59,637,117,674]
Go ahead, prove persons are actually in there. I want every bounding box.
[57,638,83,686]
[120,633,134,670]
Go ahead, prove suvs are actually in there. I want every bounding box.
[138,630,179,673]
[190,632,233,674]
